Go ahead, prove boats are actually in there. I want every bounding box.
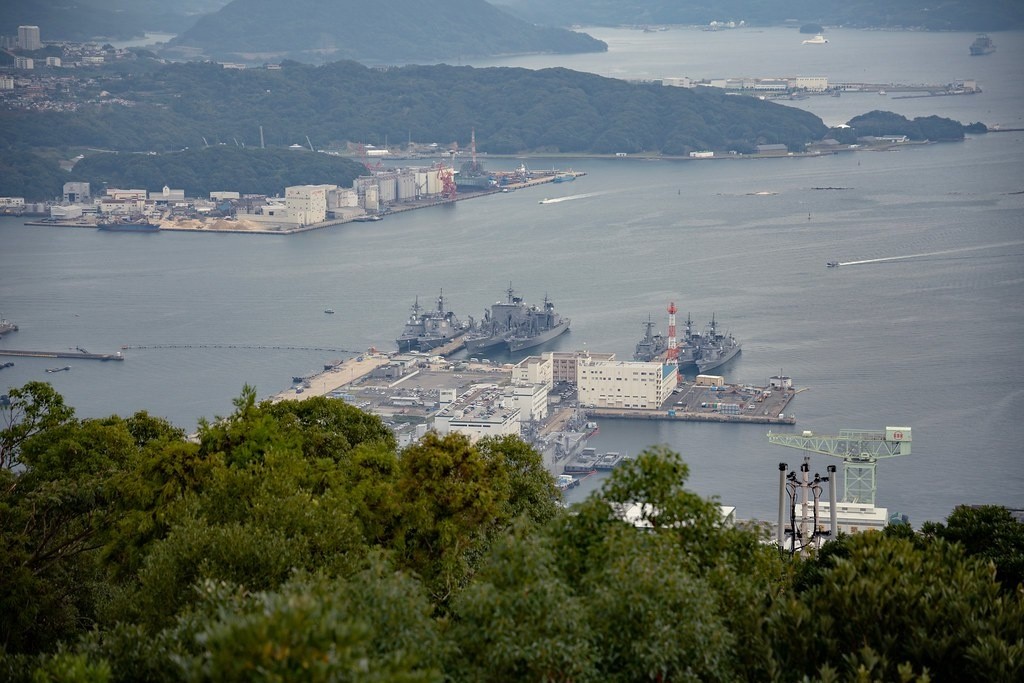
[325,308,334,313]
[632,312,668,362]
[679,311,741,373]
[396,287,470,352]
[553,177,575,182]
[463,280,571,355]
